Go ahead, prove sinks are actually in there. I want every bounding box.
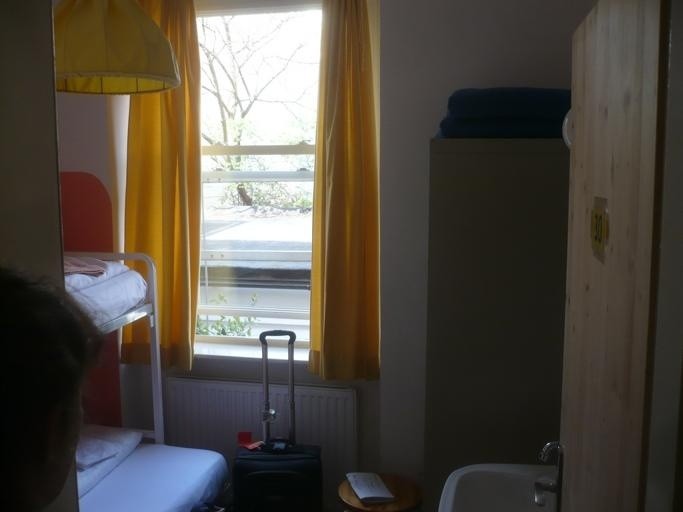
[437,464,555,512]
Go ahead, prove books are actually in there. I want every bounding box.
[348,471,395,505]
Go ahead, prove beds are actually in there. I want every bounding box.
[63,246,231,511]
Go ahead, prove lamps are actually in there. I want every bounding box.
[50,1,182,98]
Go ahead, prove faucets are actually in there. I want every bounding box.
[538,440,560,463]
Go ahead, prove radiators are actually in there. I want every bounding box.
[162,372,362,500]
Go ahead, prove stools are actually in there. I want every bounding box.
[335,470,425,511]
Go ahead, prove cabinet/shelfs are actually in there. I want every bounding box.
[423,136,571,512]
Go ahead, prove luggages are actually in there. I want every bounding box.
[232,329,323,512]
